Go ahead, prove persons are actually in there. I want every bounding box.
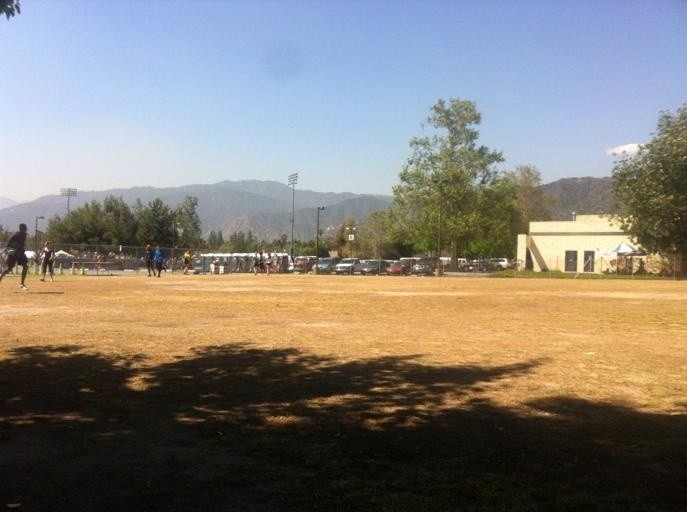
[97,251,102,268]
[0,222,28,291]
[230,249,279,277]
[181,250,194,275]
[144,244,156,278]
[108,250,116,259]
[190,250,201,261]
[622,256,633,275]
[102,252,106,269]
[633,256,646,275]
[36,239,55,282]
[152,242,166,278]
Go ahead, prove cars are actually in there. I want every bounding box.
[291,256,436,276]
[440,256,512,272]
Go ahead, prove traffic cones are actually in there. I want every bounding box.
[14,262,87,276]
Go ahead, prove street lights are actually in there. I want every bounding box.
[35,215,45,251]
[316,206,325,275]
[345,227,357,258]
